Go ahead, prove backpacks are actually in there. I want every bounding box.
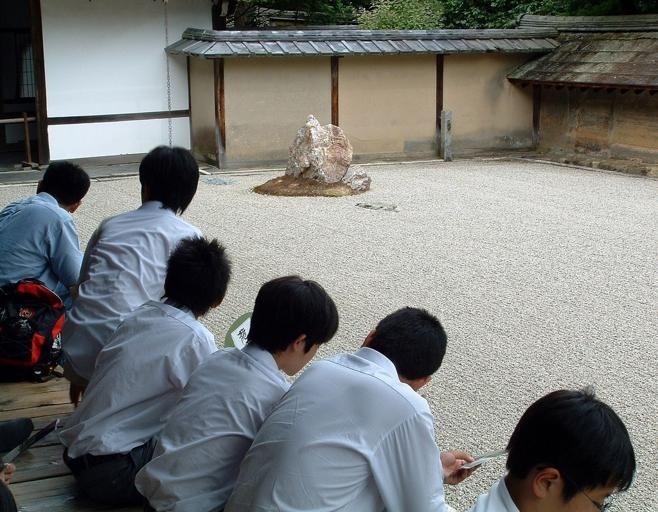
[0,278,66,382]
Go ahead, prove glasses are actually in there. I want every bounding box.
[559,470,613,512]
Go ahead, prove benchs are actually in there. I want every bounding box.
[0,372,86,511]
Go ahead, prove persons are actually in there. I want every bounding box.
[58,234,230,506]
[1,161,91,314]
[223,307,482,511]
[472,383,635,511]
[62,145,200,408]
[134,274,339,512]
[0,412,35,512]
